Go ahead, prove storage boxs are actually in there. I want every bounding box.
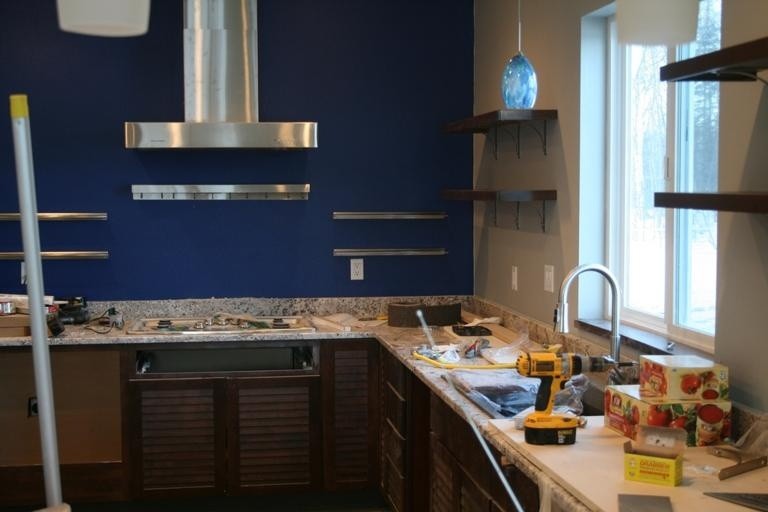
[603,355,734,447]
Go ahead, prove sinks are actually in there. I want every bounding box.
[460,377,605,423]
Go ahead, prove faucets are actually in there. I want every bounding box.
[552,264,621,363]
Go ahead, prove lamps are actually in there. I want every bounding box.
[500,1,539,110]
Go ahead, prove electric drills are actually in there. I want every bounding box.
[516,343,640,445]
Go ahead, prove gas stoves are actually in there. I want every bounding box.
[126,314,317,336]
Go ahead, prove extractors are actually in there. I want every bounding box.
[123,1,319,152]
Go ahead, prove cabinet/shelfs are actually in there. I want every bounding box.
[653,36,768,214]
[437,108,557,233]
[123,373,325,505]
[322,339,428,512]
[429,392,492,512]
[493,446,539,512]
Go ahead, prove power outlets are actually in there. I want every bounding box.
[350,258,365,281]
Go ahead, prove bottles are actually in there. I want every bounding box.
[694,404,724,448]
[702,378,720,400]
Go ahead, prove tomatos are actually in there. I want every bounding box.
[622,371,732,437]
